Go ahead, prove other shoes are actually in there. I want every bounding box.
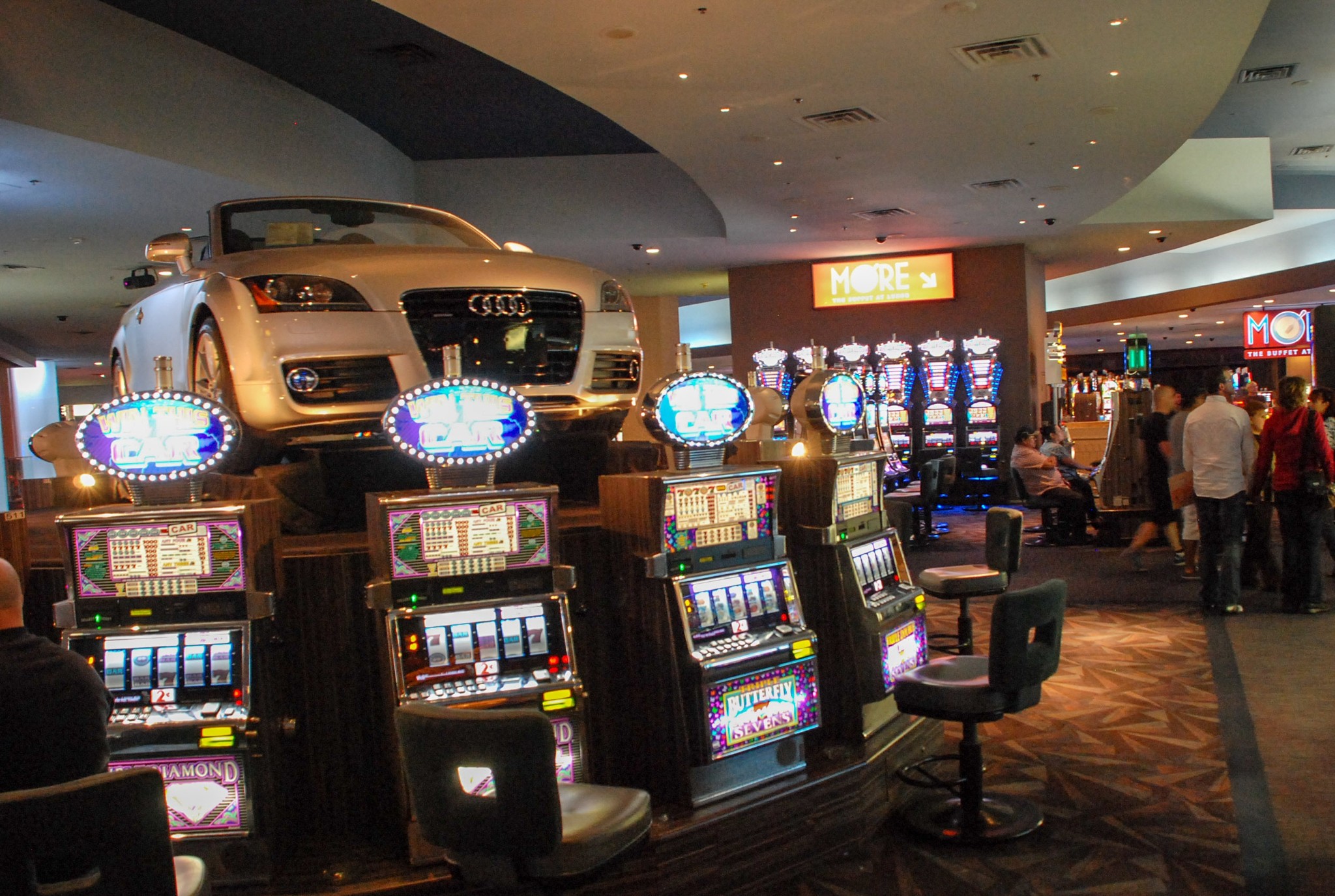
[1181,567,1202,580]
[1091,516,1107,523]
[1174,549,1187,566]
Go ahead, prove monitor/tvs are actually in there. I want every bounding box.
[1245,307,1311,356]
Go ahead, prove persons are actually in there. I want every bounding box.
[1041,424,1100,529]
[1183,362,1256,612]
[1120,382,1194,573]
[1243,399,1280,591]
[1251,375,1335,614]
[1012,426,1094,544]
[1241,378,1269,410]
[1166,386,1209,579]
[1173,392,1182,411]
[1304,386,1334,570]
[0,555,113,799]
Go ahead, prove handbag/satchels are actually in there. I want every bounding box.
[1302,469,1327,498]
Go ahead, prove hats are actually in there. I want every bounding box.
[1014,426,1040,440]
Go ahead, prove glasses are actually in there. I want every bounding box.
[1225,379,1234,383]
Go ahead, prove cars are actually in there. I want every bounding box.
[107,196,644,480]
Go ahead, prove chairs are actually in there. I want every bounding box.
[889,578,1065,844]
[395,702,652,896]
[920,503,1024,657]
[0,768,210,896]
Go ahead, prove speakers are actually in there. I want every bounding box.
[1313,305,1335,392]
